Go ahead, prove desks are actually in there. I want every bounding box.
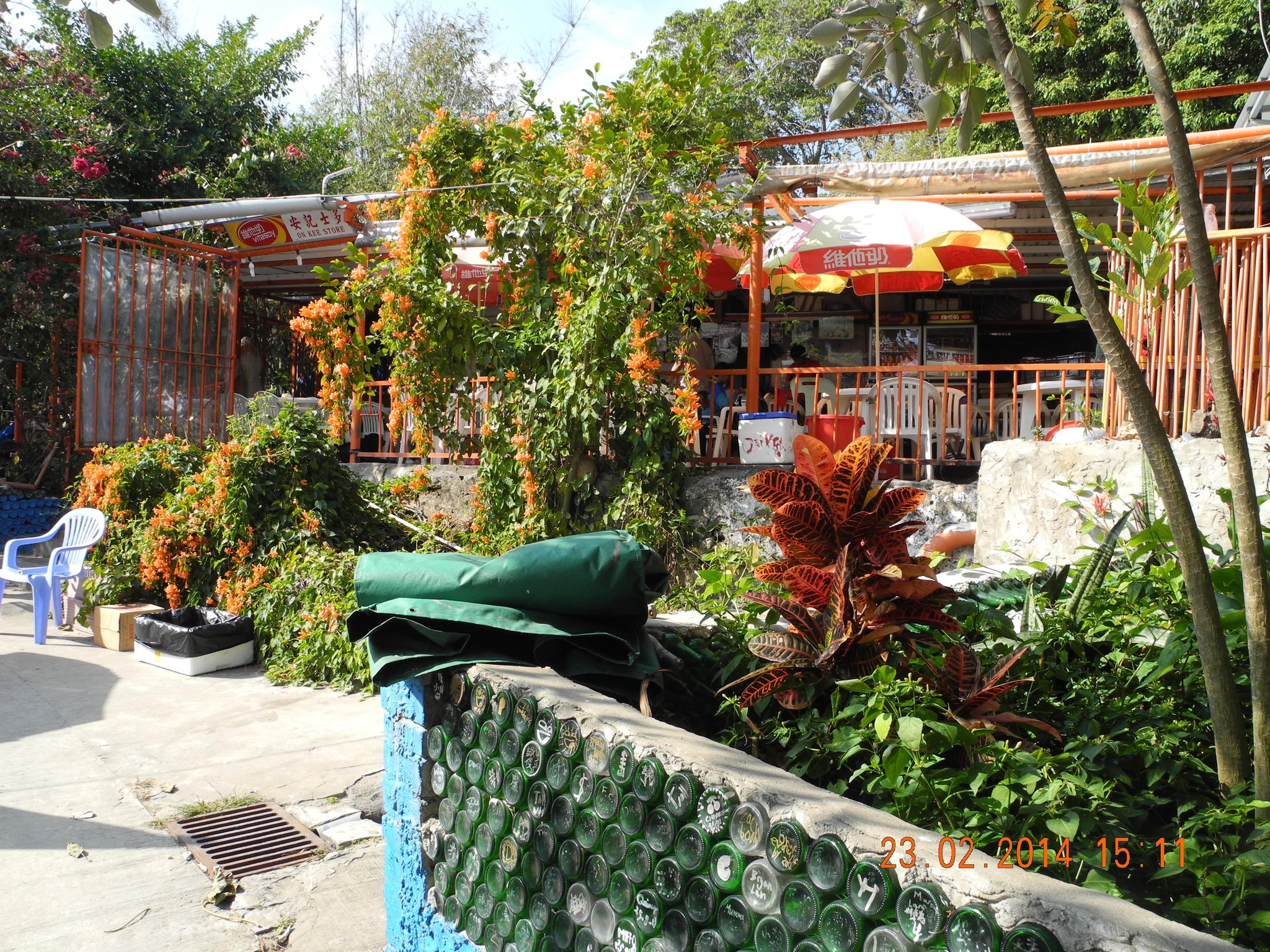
[1011,379,1106,440]
[839,387,934,455]
[246,397,320,420]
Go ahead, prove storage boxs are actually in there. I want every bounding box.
[1018,351,1106,384]
[94,603,165,652]
[134,603,255,676]
[735,411,802,465]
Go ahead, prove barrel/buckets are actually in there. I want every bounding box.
[805,414,865,454]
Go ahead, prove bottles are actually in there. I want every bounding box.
[417,664,1068,952]
[880,352,918,379]
[926,352,974,362]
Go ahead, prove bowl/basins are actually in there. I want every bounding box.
[281,393,292,398]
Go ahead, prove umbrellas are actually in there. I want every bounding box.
[658,199,1027,392]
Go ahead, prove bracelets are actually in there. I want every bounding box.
[738,389,741,394]
[663,380,669,385]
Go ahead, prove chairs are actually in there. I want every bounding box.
[0,508,106,644]
[185,376,1103,483]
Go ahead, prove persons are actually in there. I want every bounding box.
[662,314,715,456]
[784,343,824,421]
[701,362,746,464]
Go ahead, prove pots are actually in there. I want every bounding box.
[934,438,980,485]
